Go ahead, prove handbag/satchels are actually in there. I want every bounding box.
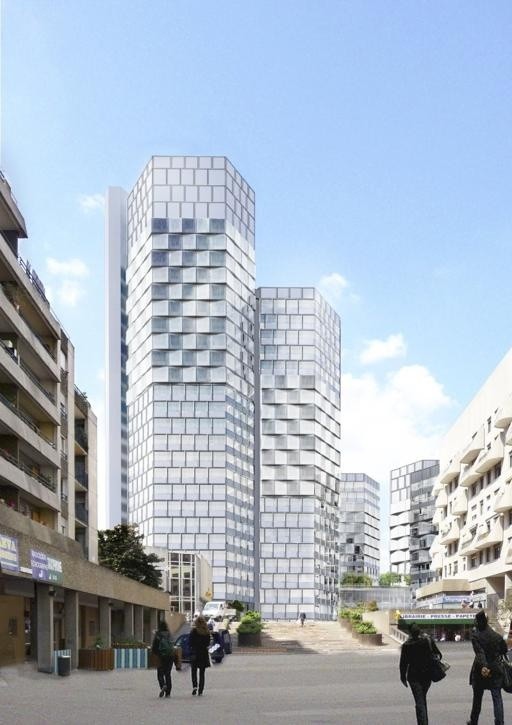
[414,636,451,682]
[493,636,512,694]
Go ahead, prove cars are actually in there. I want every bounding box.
[171,631,224,665]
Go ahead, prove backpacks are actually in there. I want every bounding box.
[155,630,175,660]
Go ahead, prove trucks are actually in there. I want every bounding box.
[200,600,238,622]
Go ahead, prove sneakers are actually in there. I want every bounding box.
[197,692,203,696]
[192,688,197,695]
[165,692,170,698]
[159,684,167,697]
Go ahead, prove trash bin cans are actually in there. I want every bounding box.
[57,655,71,676]
[24,643,32,655]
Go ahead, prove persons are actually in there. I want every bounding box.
[297,610,307,629]
[151,618,176,699]
[461,600,482,609]
[189,615,211,696]
[466,611,508,725]
[399,622,444,725]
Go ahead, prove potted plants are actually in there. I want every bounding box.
[339,607,383,645]
[236,611,264,646]
